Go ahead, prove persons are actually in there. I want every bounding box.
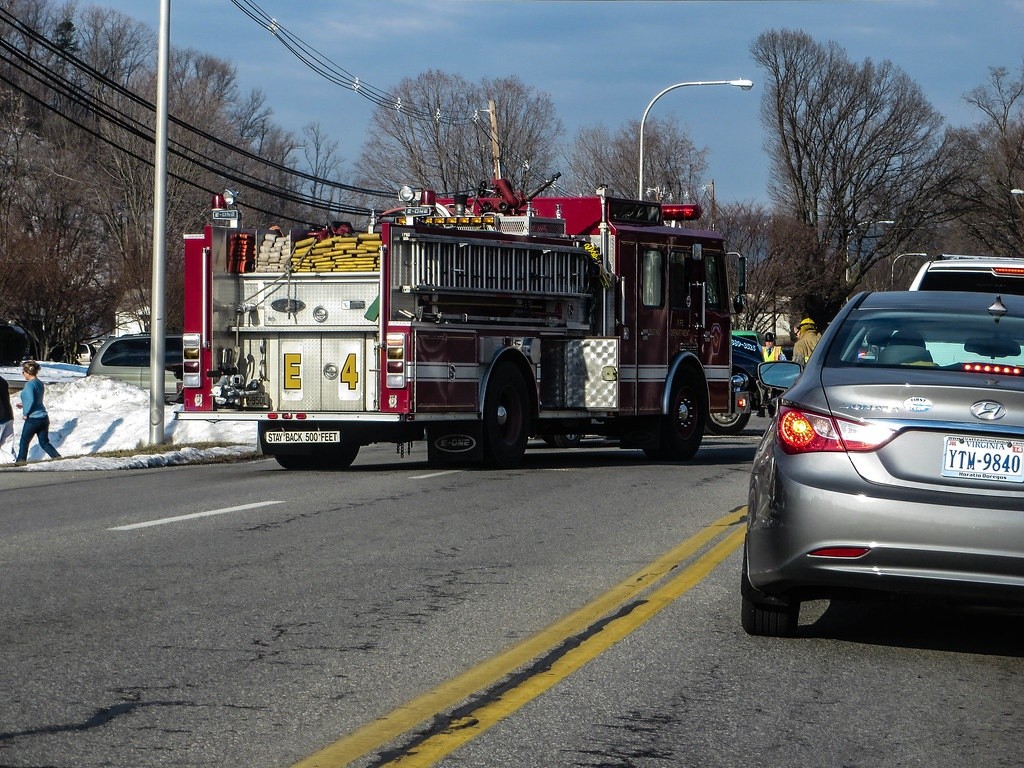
[0,376,17,464]
[793,322,800,339]
[756,332,787,417]
[14,359,61,464]
[792,318,822,370]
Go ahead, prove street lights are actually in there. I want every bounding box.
[638,77,755,202]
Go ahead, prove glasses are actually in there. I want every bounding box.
[795,330,800,336]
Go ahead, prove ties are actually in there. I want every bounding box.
[768,348,771,356]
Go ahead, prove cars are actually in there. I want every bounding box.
[86,332,185,404]
[741,290,1024,639]
[73,343,96,367]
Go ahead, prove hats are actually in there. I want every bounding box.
[22,362,41,376]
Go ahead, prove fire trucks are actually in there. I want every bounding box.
[174,172,748,471]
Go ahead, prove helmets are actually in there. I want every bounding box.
[765,332,773,342]
[800,318,815,334]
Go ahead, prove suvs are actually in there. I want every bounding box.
[878,254,1024,374]
[699,329,765,435]
